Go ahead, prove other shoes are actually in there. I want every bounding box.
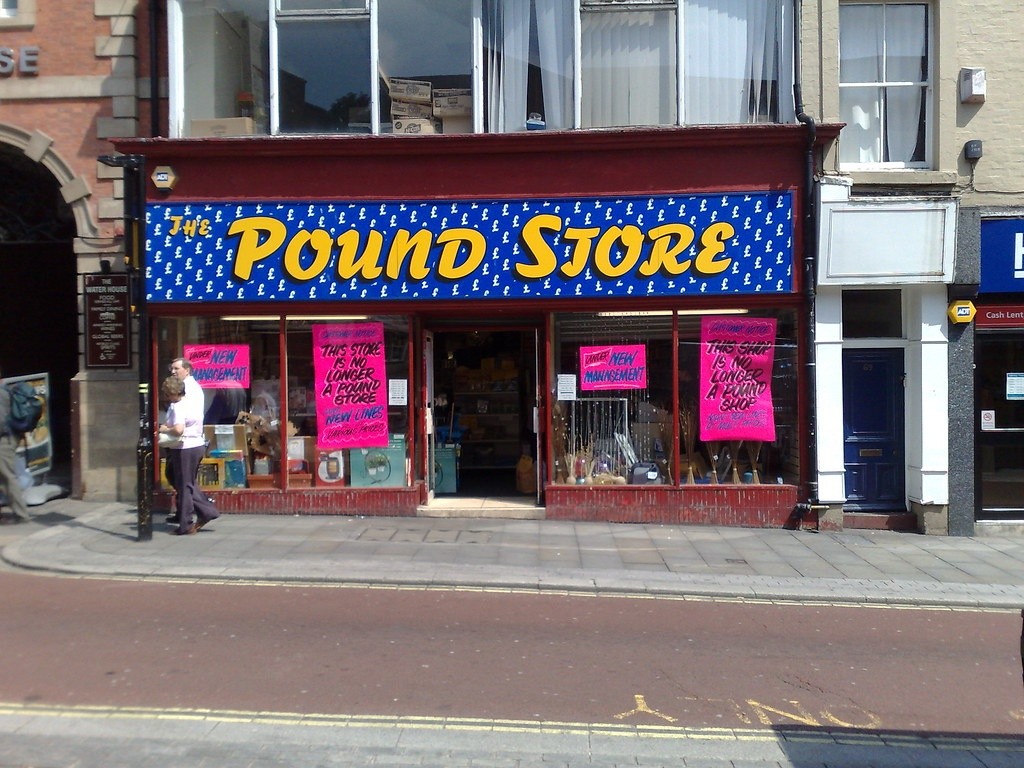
[166,515,193,523]
[1,515,23,524]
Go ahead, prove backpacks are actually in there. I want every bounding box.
[0,382,42,438]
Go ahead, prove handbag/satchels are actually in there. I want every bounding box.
[156,414,183,448]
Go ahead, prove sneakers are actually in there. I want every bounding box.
[194,513,221,530]
[169,524,197,535]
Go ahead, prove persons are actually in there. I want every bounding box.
[0,382,43,525]
[203,378,277,426]
[154,358,220,536]
